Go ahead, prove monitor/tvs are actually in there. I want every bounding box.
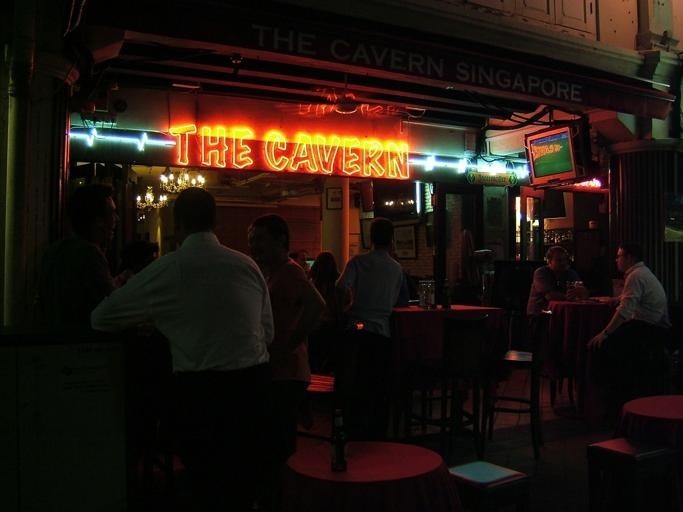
[527,126,577,185]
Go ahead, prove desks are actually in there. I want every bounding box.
[284,441,462,512]
[617,393,683,512]
[392,303,505,449]
[547,299,609,407]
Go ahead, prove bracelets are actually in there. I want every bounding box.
[601,330,609,338]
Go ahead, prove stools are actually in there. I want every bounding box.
[586,438,678,512]
[448,461,530,512]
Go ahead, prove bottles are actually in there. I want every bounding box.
[419,279,450,309]
[332,409,347,473]
[565,280,585,302]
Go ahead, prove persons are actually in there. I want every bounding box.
[527,246,589,343]
[588,243,671,348]
[47,184,407,407]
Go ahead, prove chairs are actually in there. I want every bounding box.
[476,310,553,460]
